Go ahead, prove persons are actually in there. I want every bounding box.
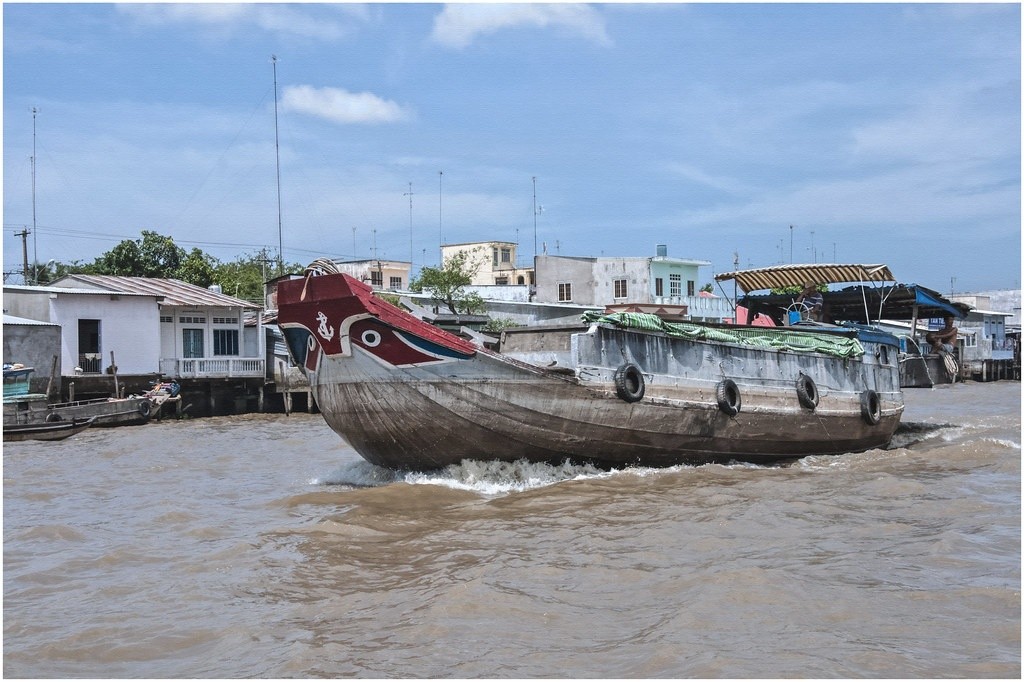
[926,312,957,354]
[797,281,823,322]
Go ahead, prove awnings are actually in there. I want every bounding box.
[713,263,898,329]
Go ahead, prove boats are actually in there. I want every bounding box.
[3,361,170,427]
[3,414,99,443]
[273,272,908,472]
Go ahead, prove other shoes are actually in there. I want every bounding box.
[940,345,954,353]
